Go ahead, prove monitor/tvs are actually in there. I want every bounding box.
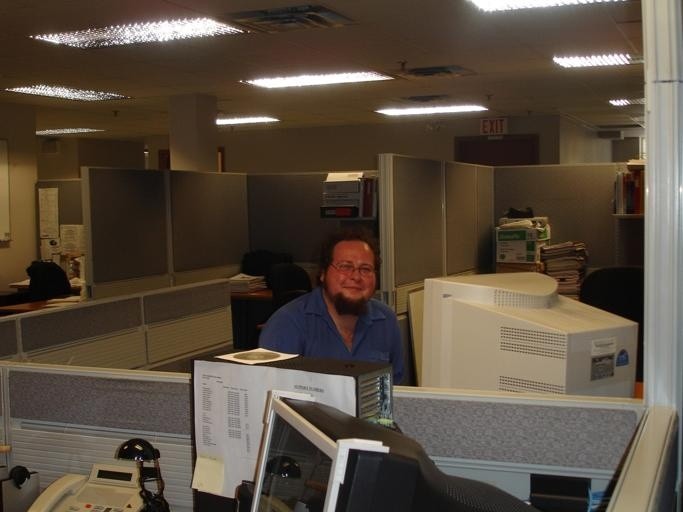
[420,270,640,399]
[248,388,537,512]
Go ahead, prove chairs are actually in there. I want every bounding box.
[26,260,71,300]
[270,261,310,308]
[579,264,642,381]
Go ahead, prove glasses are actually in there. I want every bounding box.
[328,262,377,277]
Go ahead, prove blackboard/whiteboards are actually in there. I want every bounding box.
[0,138,13,243]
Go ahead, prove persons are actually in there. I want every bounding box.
[257,225,412,387]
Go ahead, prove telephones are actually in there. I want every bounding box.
[27,463,146,512]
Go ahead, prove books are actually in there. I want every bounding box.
[228,273,267,295]
[494,214,588,299]
[611,157,645,214]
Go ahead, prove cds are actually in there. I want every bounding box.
[232,352,280,360]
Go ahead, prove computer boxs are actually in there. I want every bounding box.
[189,347,393,510]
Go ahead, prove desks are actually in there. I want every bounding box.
[0,279,80,312]
